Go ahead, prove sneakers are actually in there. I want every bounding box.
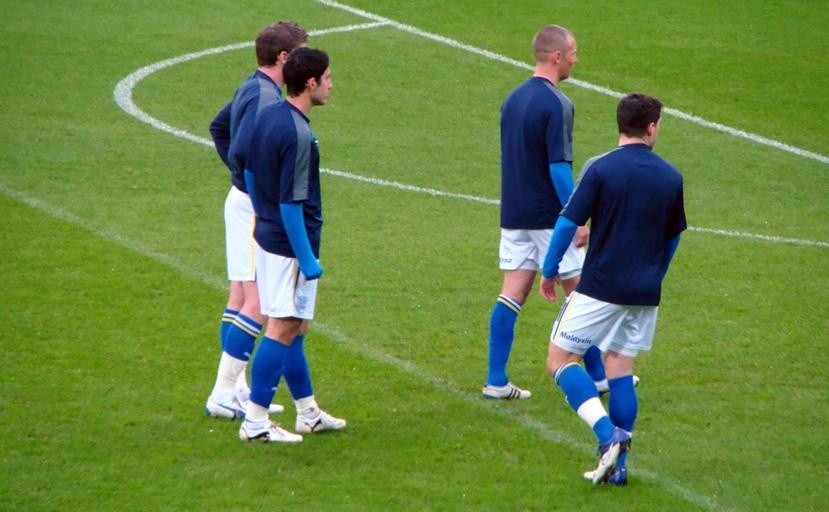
[584,427,631,485]
[482,384,534,401]
[296,410,345,434]
[594,375,638,396]
[203,395,283,419]
[239,417,303,444]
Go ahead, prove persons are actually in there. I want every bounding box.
[479,23,641,402]
[236,46,348,445]
[538,92,689,486]
[203,20,313,421]
